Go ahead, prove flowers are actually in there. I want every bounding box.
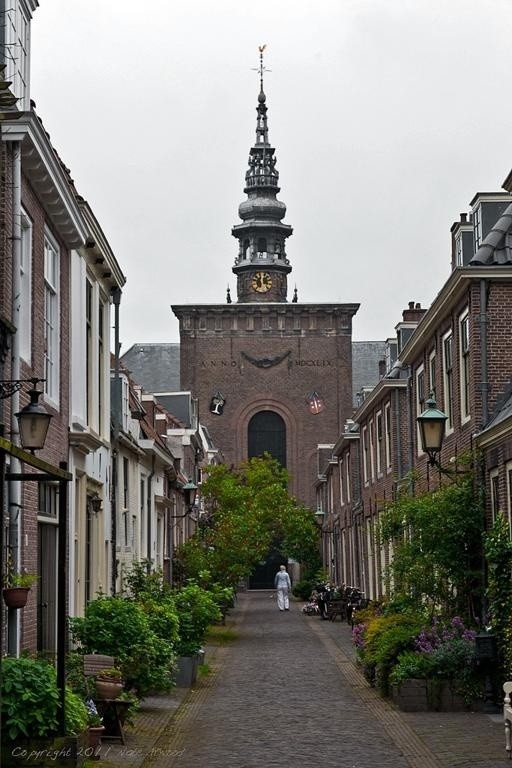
[352,602,478,712]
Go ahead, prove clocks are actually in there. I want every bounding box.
[251,272,272,293]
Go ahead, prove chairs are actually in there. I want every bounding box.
[322,585,370,624]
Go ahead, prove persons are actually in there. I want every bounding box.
[274,566,292,612]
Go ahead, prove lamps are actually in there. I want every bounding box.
[415,388,471,474]
[172,475,198,519]
[0,378,54,456]
[313,505,333,533]
